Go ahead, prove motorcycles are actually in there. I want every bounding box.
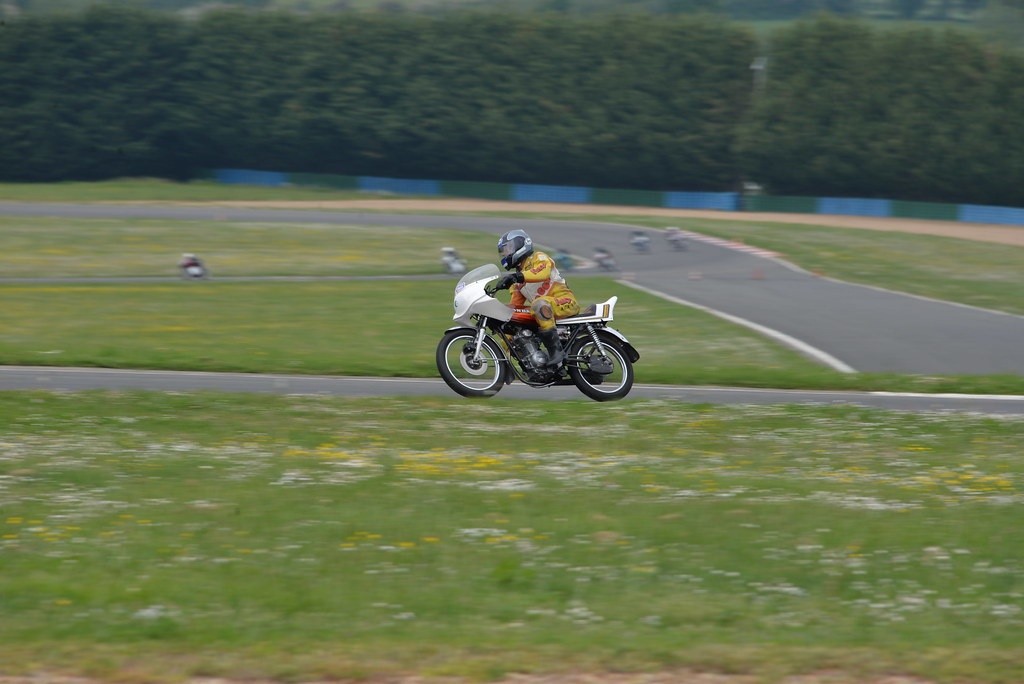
[436,264,641,402]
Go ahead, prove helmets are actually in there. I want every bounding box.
[498,230,534,271]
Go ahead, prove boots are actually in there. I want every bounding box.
[539,328,567,368]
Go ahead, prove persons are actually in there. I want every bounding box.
[496,229,580,366]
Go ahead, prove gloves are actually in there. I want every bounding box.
[496,272,524,290]
